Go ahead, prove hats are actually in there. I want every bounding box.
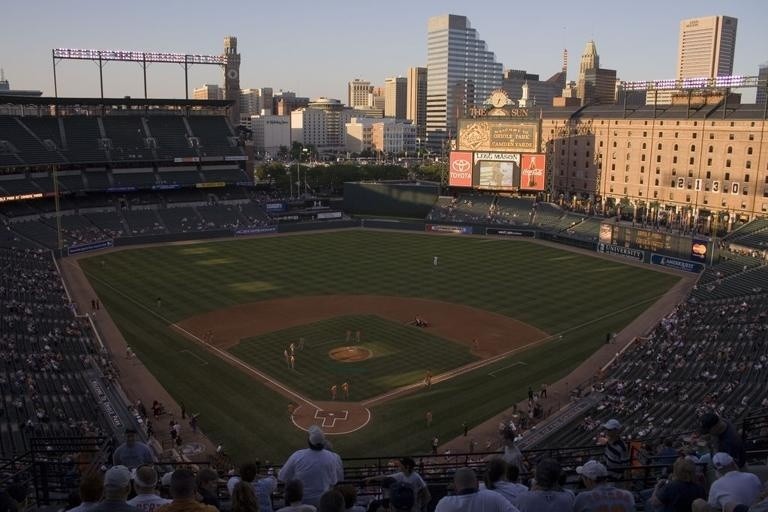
[602,419,621,429]
[104,465,172,488]
[454,467,476,489]
[309,425,324,446]
[576,460,607,480]
[713,452,733,470]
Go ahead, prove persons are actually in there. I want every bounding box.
[136,400,200,453]
[431,431,441,454]
[431,256,439,273]
[497,401,545,462]
[341,381,349,401]
[329,384,337,401]
[283,333,305,369]
[0,219,155,506]
[278,426,344,508]
[415,313,421,328]
[463,419,468,437]
[3,124,275,246]
[111,427,152,502]
[80,441,576,512]
[500,266,768,399]
[575,453,768,512]
[156,297,162,308]
[575,399,768,482]
[424,368,432,392]
[425,408,432,428]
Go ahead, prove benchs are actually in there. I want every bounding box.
[0,248,115,512]
[0,116,350,247]
[427,190,603,242]
[520,217,768,460]
